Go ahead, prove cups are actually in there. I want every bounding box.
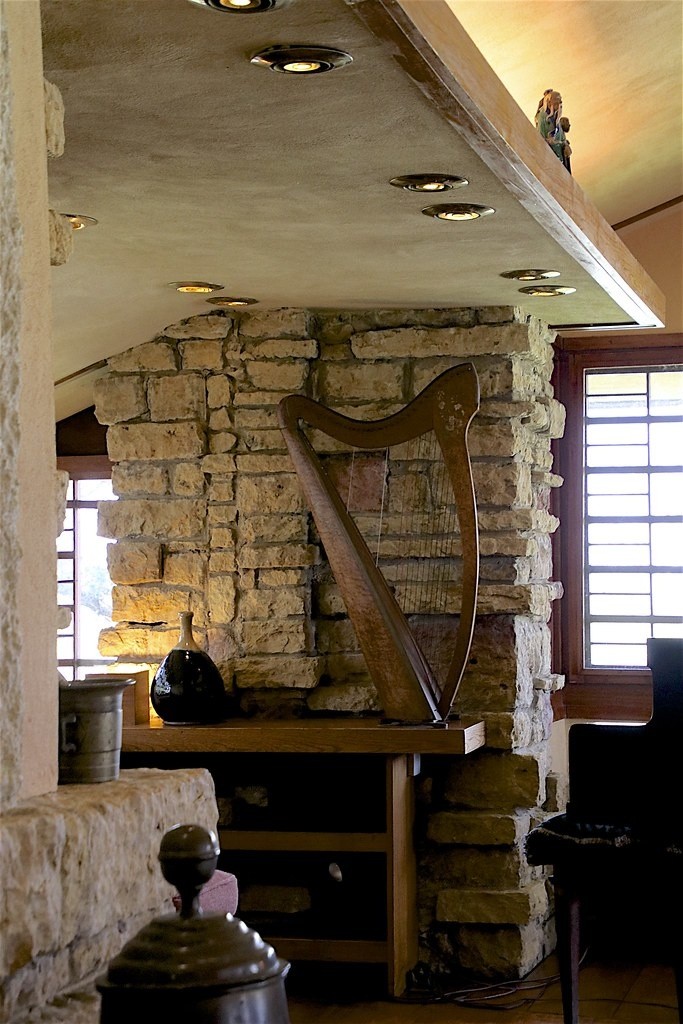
[59,678,137,783]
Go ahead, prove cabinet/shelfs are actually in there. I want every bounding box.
[120,717,487,1001]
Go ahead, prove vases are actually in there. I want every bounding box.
[151,611,226,726]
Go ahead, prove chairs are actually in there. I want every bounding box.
[524,724,683,1023]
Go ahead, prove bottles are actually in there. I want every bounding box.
[150,612,226,723]
